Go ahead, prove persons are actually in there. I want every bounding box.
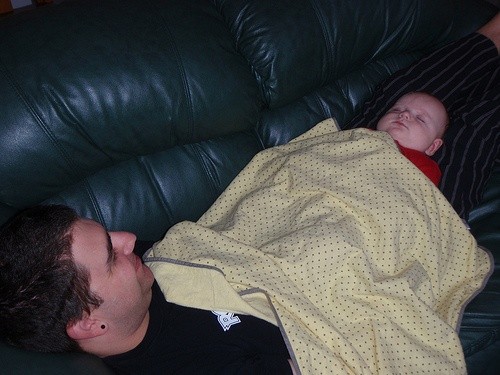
[369,92,448,187]
[1,15,500,375]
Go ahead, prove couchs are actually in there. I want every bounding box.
[0,0,500,375]
